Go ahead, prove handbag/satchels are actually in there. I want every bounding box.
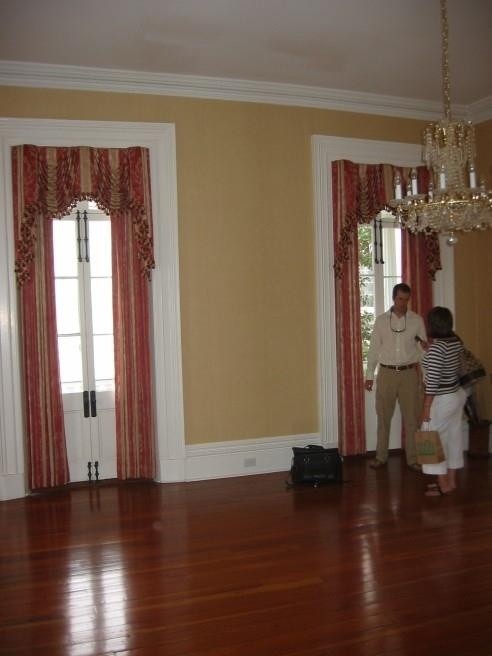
[413,418,445,466]
[420,420,470,451]
[455,333,487,389]
[288,442,345,488]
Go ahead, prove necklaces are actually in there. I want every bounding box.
[390,306,407,333]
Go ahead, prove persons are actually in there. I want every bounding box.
[365,283,427,471]
[420,306,468,497]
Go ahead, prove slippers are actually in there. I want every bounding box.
[424,481,458,498]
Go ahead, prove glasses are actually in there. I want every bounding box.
[389,314,407,333]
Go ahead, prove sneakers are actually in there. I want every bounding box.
[367,457,425,473]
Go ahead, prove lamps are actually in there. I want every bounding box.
[383,1,491,246]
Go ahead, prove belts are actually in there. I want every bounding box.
[379,361,419,371]
